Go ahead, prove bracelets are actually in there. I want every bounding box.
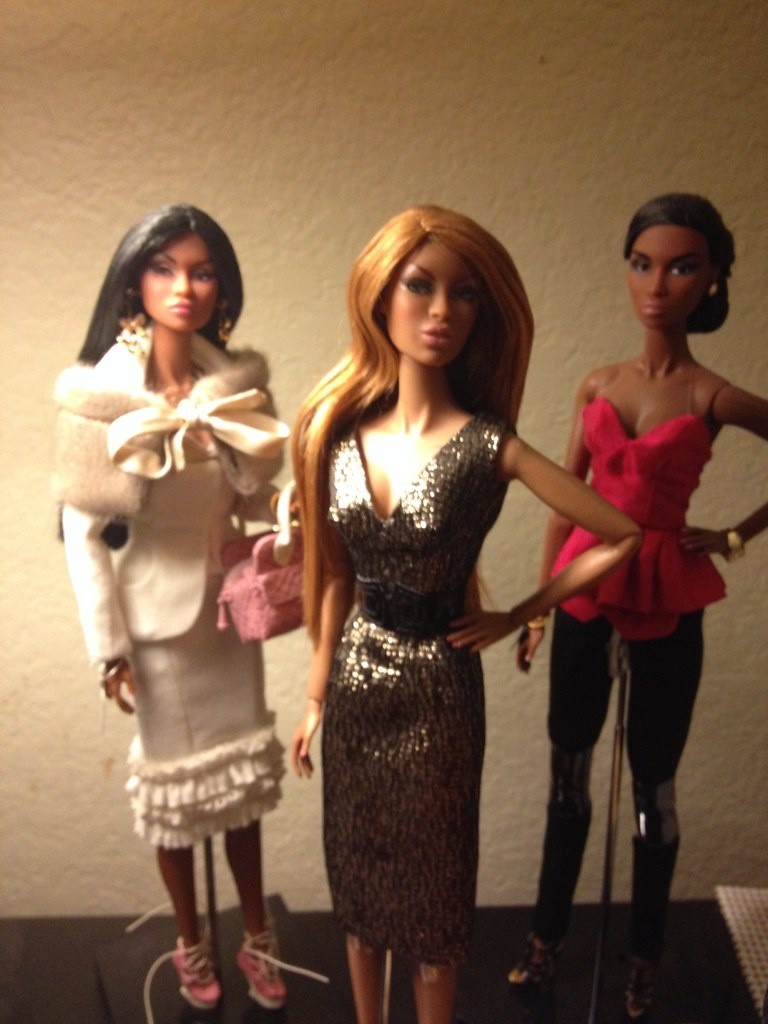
[720,526,747,564]
[97,657,125,687]
[521,610,554,631]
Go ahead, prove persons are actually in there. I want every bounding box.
[507,190,767,1019]
[289,201,645,1024]
[47,203,300,1006]
